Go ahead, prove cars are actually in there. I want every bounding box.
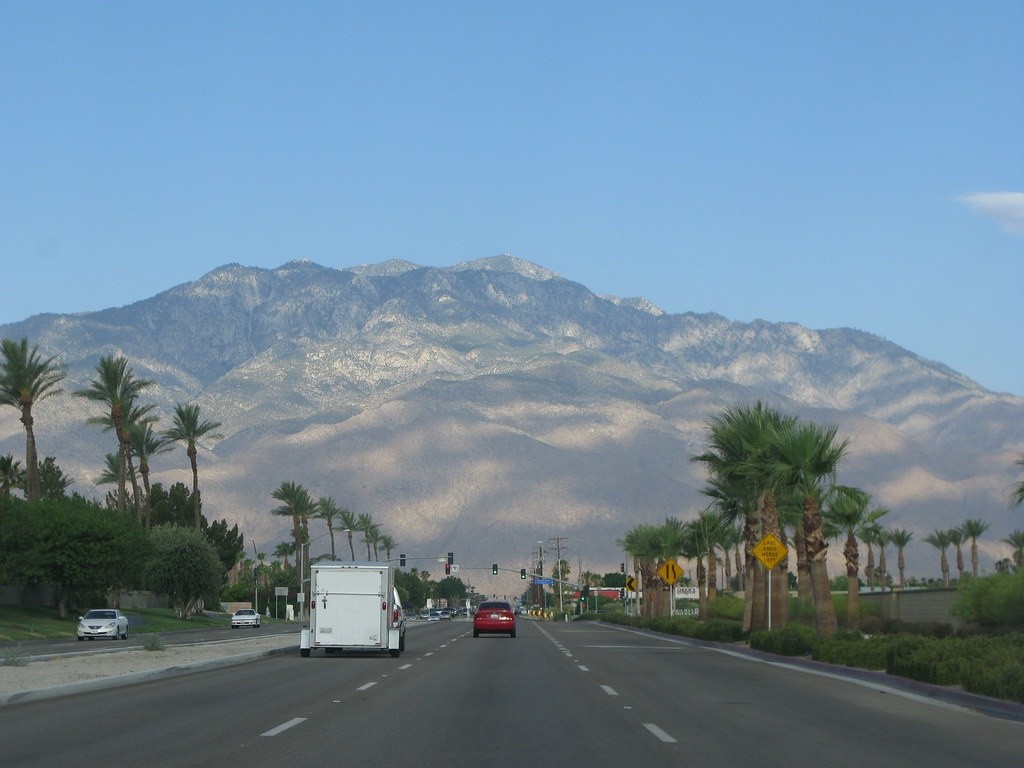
[406,607,478,623]
[77,609,129,640]
[231,609,261,629]
[472,601,516,638]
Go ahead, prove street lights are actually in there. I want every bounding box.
[300,528,351,621]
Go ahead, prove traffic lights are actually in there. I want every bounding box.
[445,563,451,575]
[621,563,624,573]
[493,564,498,575]
[520,569,527,579]
[619,589,624,599]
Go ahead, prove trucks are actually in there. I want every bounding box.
[300,559,407,658]
